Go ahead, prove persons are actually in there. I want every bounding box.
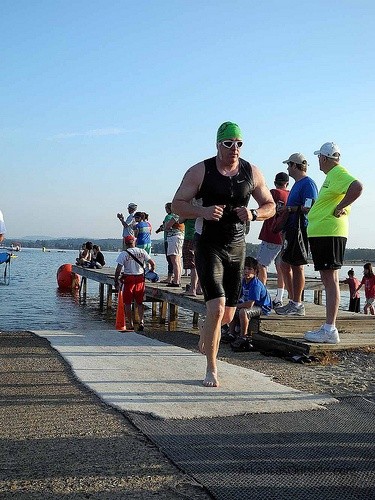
[113,203,155,331]
[222,256,272,351]
[0,210,6,241]
[255,172,290,309]
[160,197,203,296]
[275,153,319,313]
[304,141,363,343]
[343,270,361,313]
[75,241,107,270]
[353,263,375,315]
[171,121,276,386]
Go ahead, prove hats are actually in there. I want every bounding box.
[127,202,137,211]
[125,235,134,242]
[282,152,308,167]
[216,121,242,143]
[314,142,341,159]
[133,212,144,218]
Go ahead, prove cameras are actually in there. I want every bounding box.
[278,201,284,210]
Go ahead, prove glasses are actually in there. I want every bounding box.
[218,140,243,149]
[318,155,324,158]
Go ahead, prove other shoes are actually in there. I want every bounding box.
[159,279,171,283]
[138,322,144,331]
[231,334,248,347]
[166,280,182,287]
[275,301,305,316]
[303,324,339,344]
[272,300,283,309]
[129,326,134,330]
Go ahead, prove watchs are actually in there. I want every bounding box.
[250,209,258,222]
